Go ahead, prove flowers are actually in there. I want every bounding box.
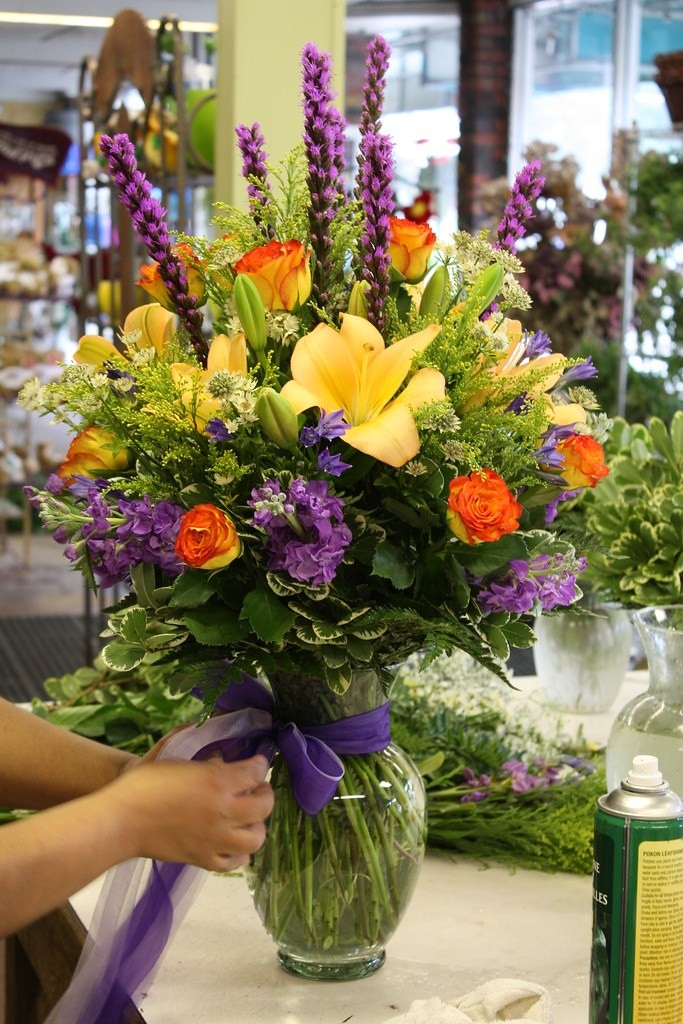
[387,669,607,876]
[18,34,609,695]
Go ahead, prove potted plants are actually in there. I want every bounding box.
[531,411,683,715]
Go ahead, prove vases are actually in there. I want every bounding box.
[605,603,683,800]
[243,667,428,980]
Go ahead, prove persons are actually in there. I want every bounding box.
[0,694,274,938]
